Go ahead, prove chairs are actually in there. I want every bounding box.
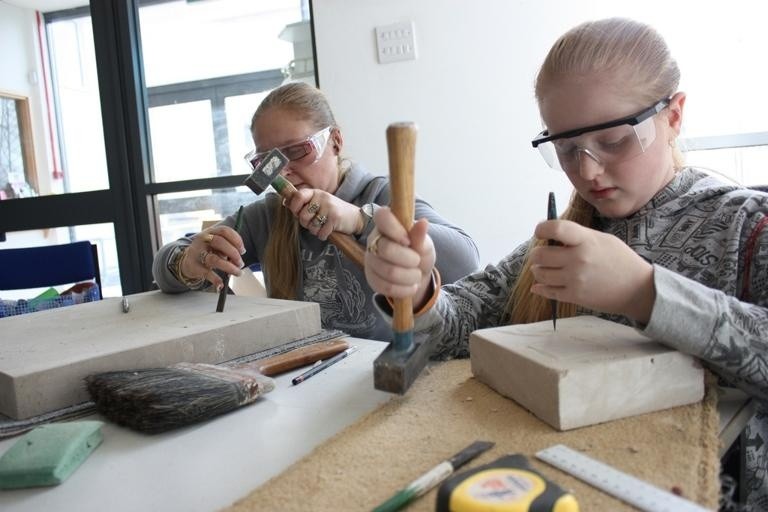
[0,239,100,317]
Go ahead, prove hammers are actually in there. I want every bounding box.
[373,121,429,397]
[243,147,366,271]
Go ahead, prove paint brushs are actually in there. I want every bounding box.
[371,440,494,512]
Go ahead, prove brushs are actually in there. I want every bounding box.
[83,339,349,436]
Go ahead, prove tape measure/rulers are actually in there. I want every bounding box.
[436,444,711,512]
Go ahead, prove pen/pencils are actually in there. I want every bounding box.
[292,346,358,385]
[122,296,129,313]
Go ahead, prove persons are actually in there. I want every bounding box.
[152,82,480,341]
[363,19,767,512]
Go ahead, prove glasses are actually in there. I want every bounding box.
[531,100,671,172]
[245,125,332,169]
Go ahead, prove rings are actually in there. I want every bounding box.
[204,234,214,247]
[368,232,382,254]
[199,250,209,265]
[308,201,327,227]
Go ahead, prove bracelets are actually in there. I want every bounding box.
[385,267,441,318]
[175,247,205,290]
